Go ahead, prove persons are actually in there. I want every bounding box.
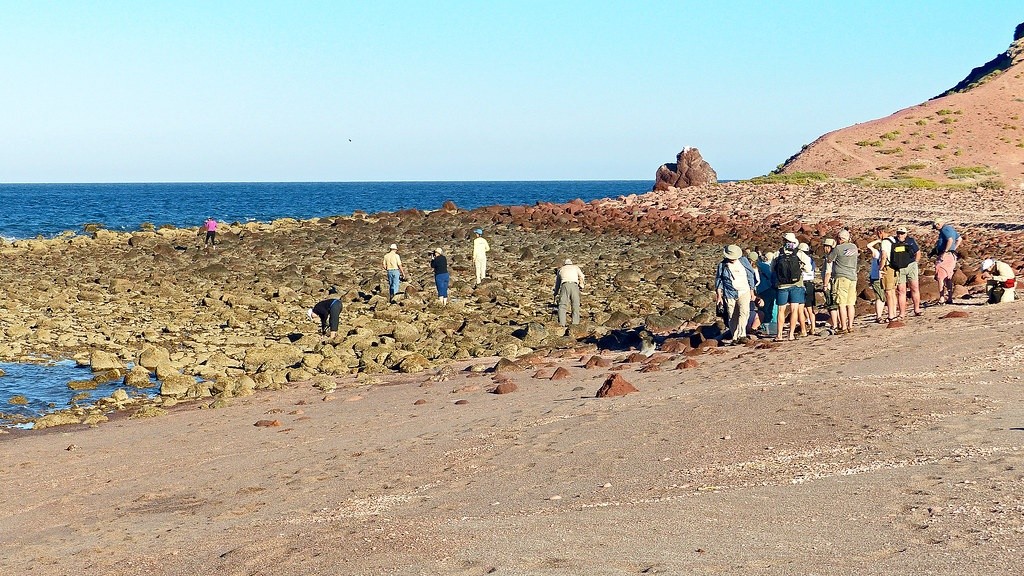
[742,233,820,343]
[716,245,756,346]
[932,218,962,306]
[553,258,584,327]
[867,240,886,324]
[827,230,858,334]
[431,248,449,307]
[894,227,924,320]
[981,259,1017,302]
[383,244,405,300]
[877,225,898,323]
[205,217,218,250]
[308,299,343,339]
[473,229,490,285]
[822,239,842,335]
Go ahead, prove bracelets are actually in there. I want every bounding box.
[944,250,948,253]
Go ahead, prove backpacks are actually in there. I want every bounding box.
[882,237,910,269]
[774,248,801,284]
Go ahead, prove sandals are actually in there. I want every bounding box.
[884,317,898,322]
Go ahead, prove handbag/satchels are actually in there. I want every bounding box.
[989,285,1005,303]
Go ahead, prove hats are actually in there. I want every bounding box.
[563,259,573,264]
[435,248,443,254]
[981,259,994,271]
[474,229,483,235]
[782,232,796,243]
[823,239,836,246]
[389,243,398,250]
[799,243,810,250]
[307,308,313,320]
[897,228,908,233]
[723,245,742,260]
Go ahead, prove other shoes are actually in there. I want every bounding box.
[946,300,952,304]
[838,328,855,334]
[934,302,945,306]
[730,337,746,346]
[771,336,799,342]
[876,317,884,324]
